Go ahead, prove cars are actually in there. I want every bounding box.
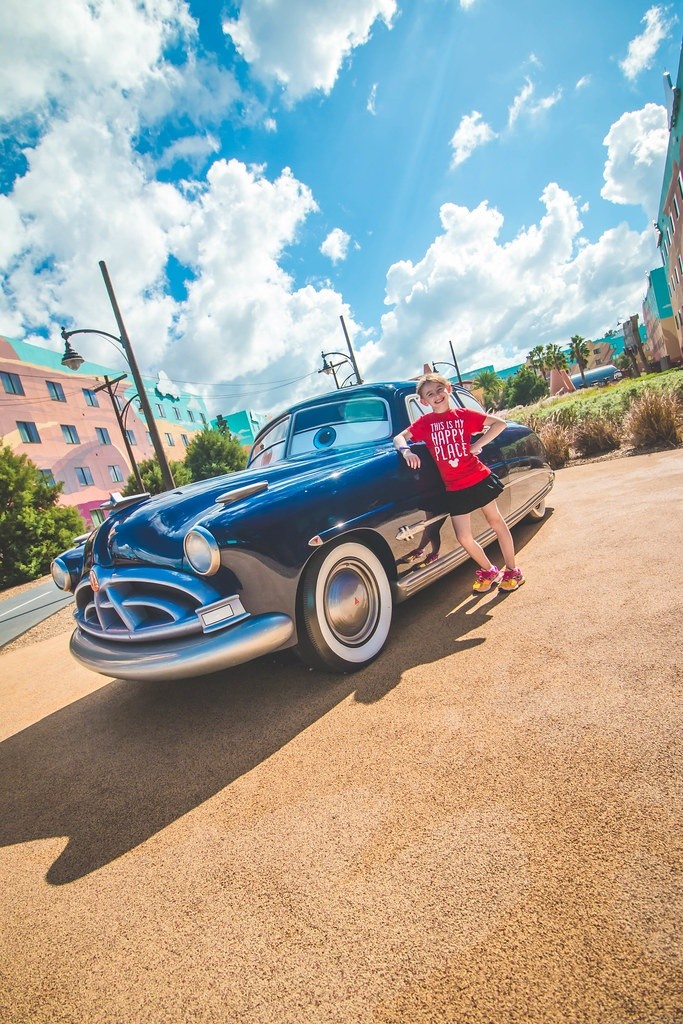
[48,379,554,673]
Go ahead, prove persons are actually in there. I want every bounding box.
[394,373,526,592]
[400,451,447,571]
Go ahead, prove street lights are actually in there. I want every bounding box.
[432,361,464,388]
[322,352,363,385]
[119,393,146,491]
[60,327,174,494]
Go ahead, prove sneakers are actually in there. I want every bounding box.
[472,566,501,592]
[417,552,439,568]
[498,567,525,593]
[402,549,424,563]
